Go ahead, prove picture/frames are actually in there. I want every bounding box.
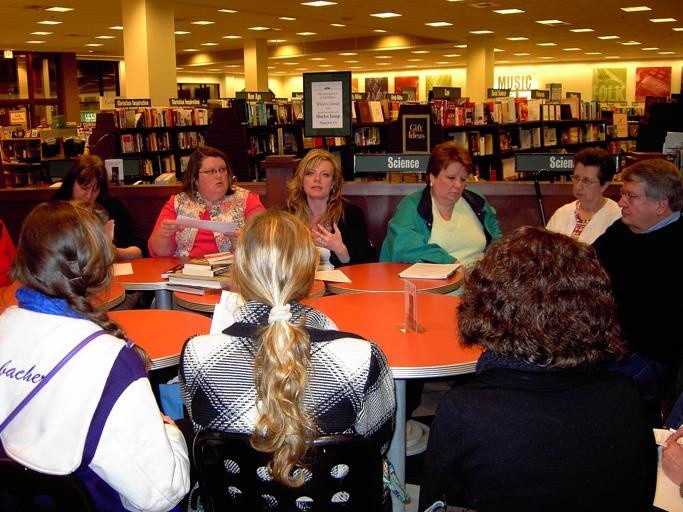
[302,72,354,138]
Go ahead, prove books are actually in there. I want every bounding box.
[1,82,621,180]
[160,251,235,297]
[397,262,462,279]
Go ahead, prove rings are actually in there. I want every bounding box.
[316,238,321,244]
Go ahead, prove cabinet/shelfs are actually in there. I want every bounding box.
[0,95,683,183]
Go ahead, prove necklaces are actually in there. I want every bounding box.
[577,202,602,224]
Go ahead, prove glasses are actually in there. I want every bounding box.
[570,174,597,184]
[619,187,640,200]
[199,166,227,175]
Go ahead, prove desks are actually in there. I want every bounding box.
[0,255,492,512]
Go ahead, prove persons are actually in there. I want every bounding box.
[589,159,682,430]
[661,426,683,498]
[376,140,507,266]
[282,147,368,266]
[535,146,617,244]
[177,208,395,463]
[415,222,657,511]
[143,143,268,260]
[0,199,192,512]
[41,153,154,310]
